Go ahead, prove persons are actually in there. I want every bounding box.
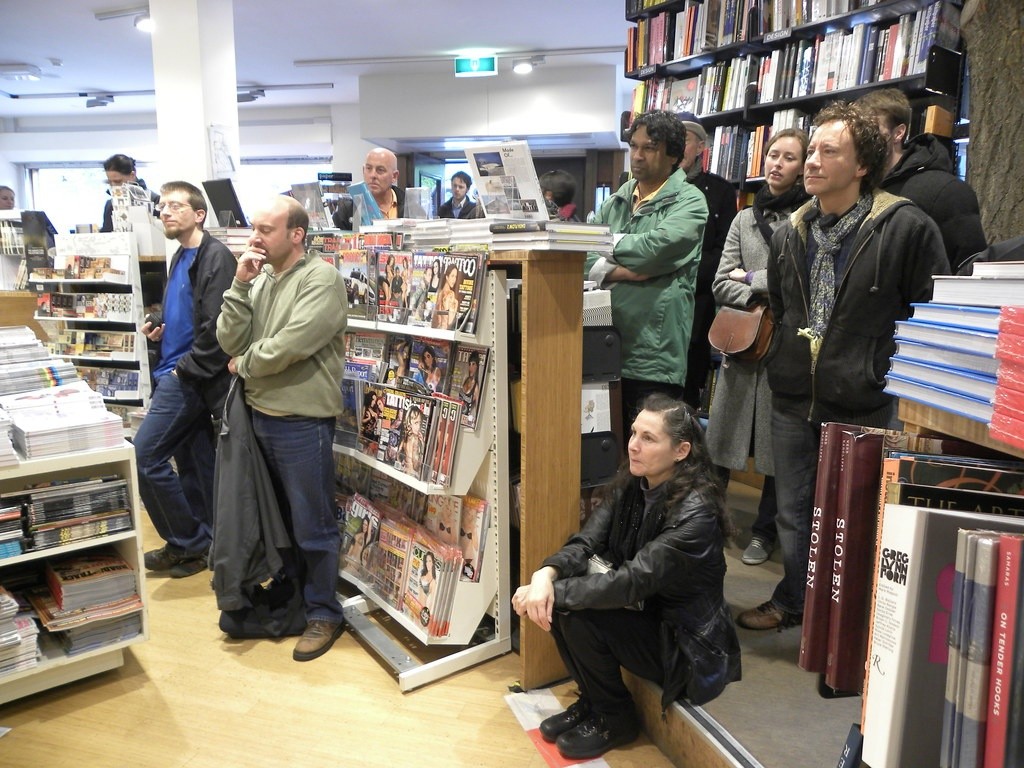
[735,101,949,632]
[704,127,814,566]
[434,265,462,329]
[534,169,581,222]
[667,112,738,409]
[94,153,162,233]
[437,171,486,219]
[333,148,430,229]
[582,108,709,455]
[343,517,372,574]
[511,391,743,761]
[414,551,437,621]
[134,181,237,580]
[396,404,424,475]
[459,351,480,419]
[207,195,350,663]
[850,87,987,276]
[0,185,16,210]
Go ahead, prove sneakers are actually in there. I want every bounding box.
[168,552,207,579]
[293,614,345,662]
[144,541,199,571]
[737,600,802,631]
[556,705,643,759]
[539,694,593,742]
[741,536,775,564]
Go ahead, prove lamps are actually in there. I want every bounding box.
[86,93,114,107]
[238,88,263,103]
[514,53,545,73]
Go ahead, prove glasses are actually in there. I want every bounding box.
[105,178,121,184]
[154,201,190,210]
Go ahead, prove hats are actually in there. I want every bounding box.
[676,111,708,141]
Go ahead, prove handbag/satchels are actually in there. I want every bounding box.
[708,306,779,365]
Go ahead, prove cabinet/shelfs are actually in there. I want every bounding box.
[0,440,151,711]
[619,0,963,207]
[31,230,151,408]
[333,266,509,691]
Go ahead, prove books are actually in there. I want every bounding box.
[798,259,1024,768]
[0,140,615,691]
[628,0,971,214]
